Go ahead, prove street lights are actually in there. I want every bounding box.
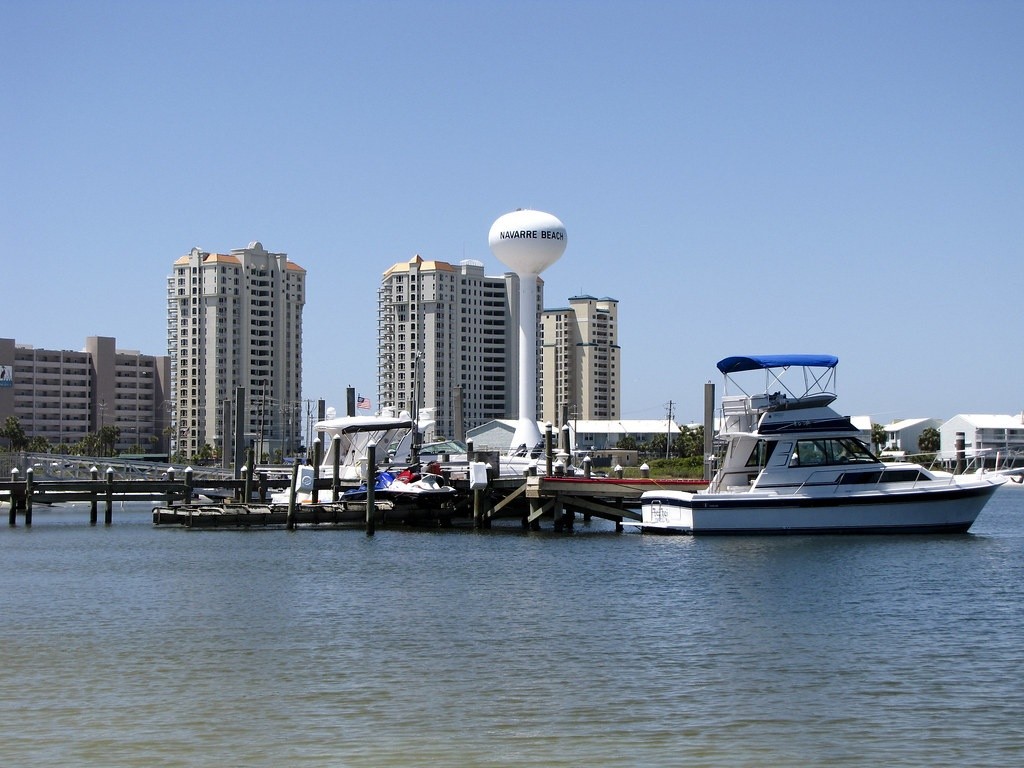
[99,400,108,452]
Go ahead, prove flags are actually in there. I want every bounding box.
[357,397,371,409]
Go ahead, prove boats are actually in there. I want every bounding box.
[978,466,1024,484]
[270,407,607,506]
[618,353,1024,535]
